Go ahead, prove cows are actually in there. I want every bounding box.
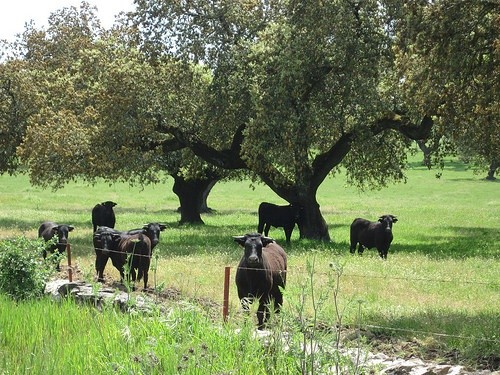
[258,202,305,244]
[92,201,117,233]
[350,215,398,260]
[38,222,74,272]
[93,223,167,290]
[233,233,287,330]
